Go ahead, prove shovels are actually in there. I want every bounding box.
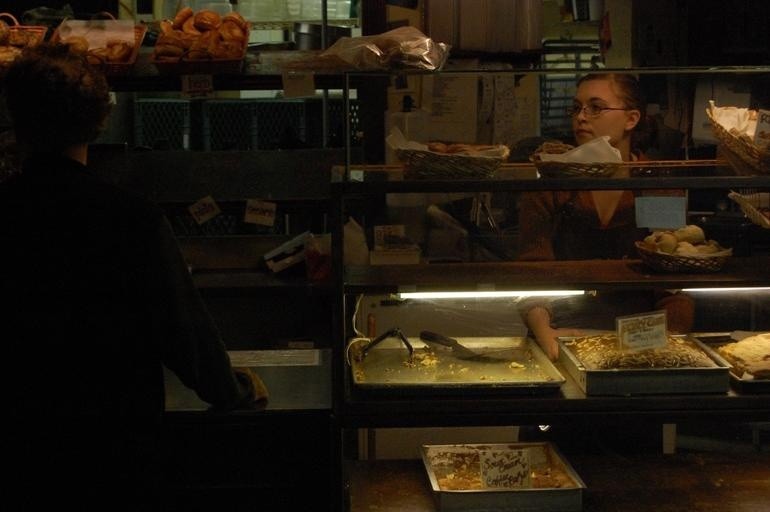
[420,331,510,359]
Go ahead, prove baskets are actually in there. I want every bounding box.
[529,143,624,177]
[395,144,511,178]
[0,12,48,64]
[635,241,733,272]
[706,106,770,173]
[150,32,248,73]
[50,12,149,65]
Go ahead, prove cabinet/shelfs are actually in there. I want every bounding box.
[106,53,769,512]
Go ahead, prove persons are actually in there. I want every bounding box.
[0,41,271,512]
[513,72,698,362]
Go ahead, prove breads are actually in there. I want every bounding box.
[0,20,39,63]
[59,36,132,61]
[155,6,249,57]
[357,25,440,71]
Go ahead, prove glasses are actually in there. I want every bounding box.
[566,105,627,117]
